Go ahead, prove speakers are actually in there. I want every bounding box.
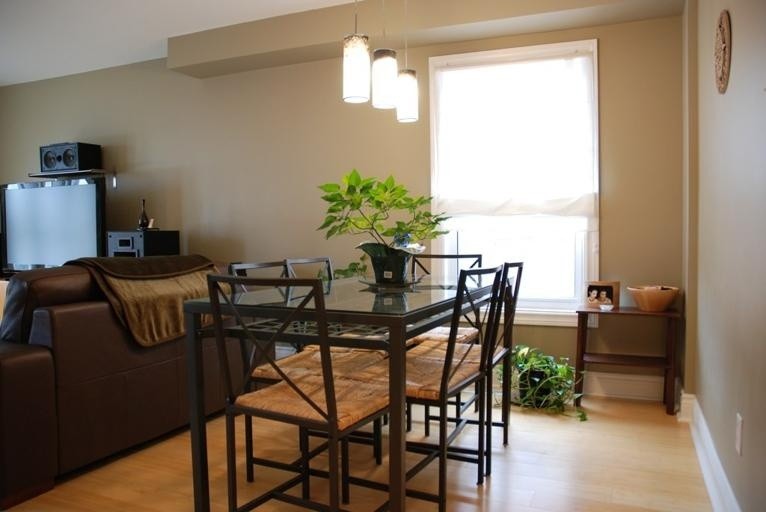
[39,141,104,175]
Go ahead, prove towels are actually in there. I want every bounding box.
[65,254,235,347]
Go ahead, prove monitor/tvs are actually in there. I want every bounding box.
[0,172,109,281]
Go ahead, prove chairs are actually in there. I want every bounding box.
[405,255,482,430]
[207,273,390,511]
[284,256,417,425]
[425,262,523,444]
[228,261,388,456]
[342,266,505,512]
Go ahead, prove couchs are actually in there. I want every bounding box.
[0,255,275,512]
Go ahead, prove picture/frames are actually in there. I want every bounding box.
[583,281,620,311]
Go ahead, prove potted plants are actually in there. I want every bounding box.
[315,168,452,288]
[495,345,589,422]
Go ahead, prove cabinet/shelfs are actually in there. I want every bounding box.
[573,306,681,415]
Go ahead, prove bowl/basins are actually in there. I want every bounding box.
[627,285,680,311]
[600,305,614,311]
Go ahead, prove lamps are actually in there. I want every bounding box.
[342,1,371,103]
[397,0,419,122]
[371,0,399,109]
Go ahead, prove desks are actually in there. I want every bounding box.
[185,276,517,512]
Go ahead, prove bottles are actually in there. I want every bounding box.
[137,199,149,227]
